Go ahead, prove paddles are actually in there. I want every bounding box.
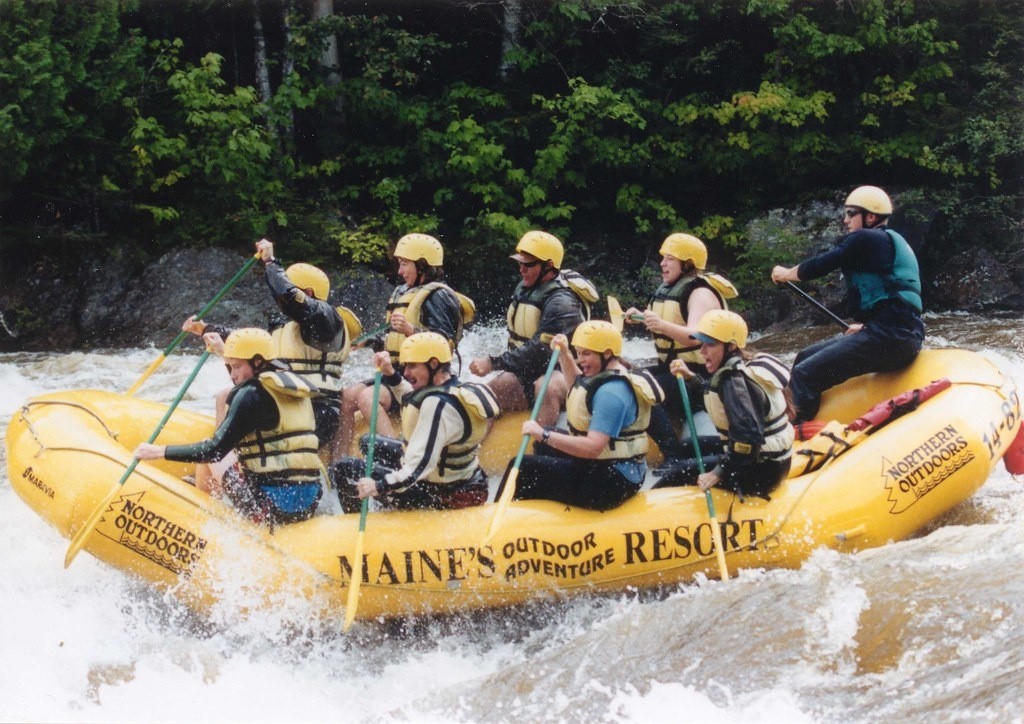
[126,251,262,393]
[63,346,212,570]
[482,345,563,546]
[605,296,645,334]
[776,277,850,330]
[340,366,383,633]
[674,361,729,588]
[351,322,394,347]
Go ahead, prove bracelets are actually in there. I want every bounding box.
[414,327,422,333]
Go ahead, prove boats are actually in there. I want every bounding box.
[5,351,1024,632]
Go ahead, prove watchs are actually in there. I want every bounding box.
[541,430,550,445]
[265,256,275,263]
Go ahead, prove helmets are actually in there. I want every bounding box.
[698,309,748,348]
[285,263,330,302]
[659,233,708,269]
[516,230,565,269]
[571,319,622,357]
[394,233,444,265]
[843,186,893,215]
[398,332,452,363]
[222,327,276,361]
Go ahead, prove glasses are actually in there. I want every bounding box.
[847,209,862,217]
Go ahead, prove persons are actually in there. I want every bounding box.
[494,320,665,511]
[334,332,500,513]
[771,185,926,425]
[649,309,795,503]
[182,237,362,450]
[132,328,333,526]
[624,232,739,477]
[334,233,475,456]
[468,230,599,447]
